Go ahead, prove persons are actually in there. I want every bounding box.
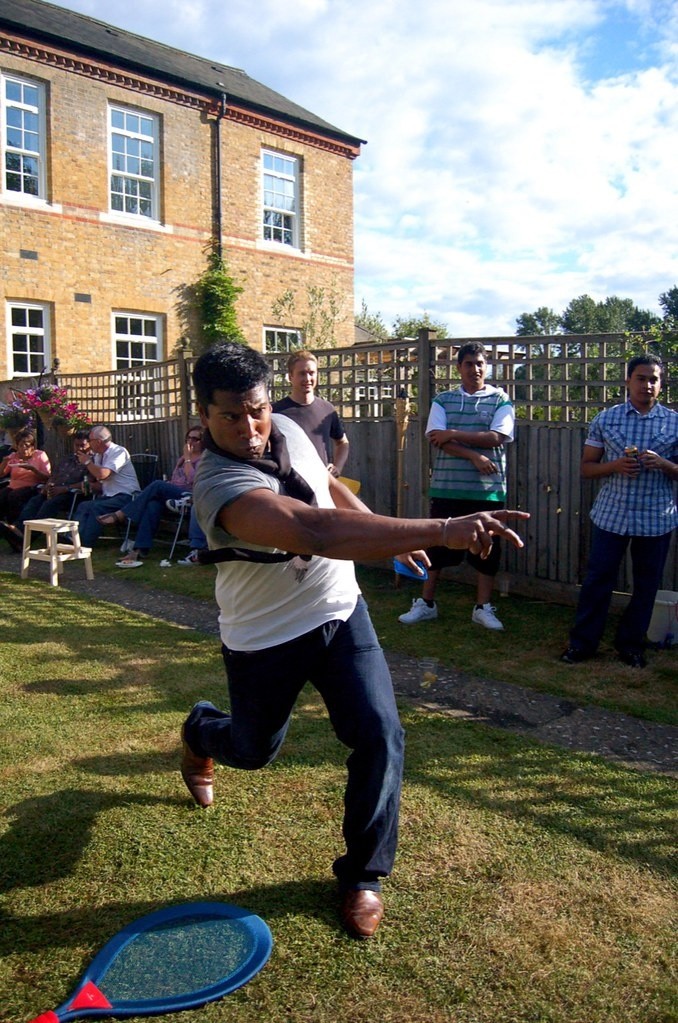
[271,352,350,477]
[0,431,53,522]
[0,430,96,553]
[560,354,678,668]
[61,425,141,548]
[177,504,208,565]
[96,425,204,561]
[179,341,530,938]
[396,341,516,629]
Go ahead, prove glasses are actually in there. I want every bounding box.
[186,436,201,442]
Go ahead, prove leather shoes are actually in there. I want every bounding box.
[344,889,384,937]
[180,723,214,808]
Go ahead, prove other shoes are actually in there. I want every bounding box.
[620,647,646,668]
[559,647,593,661]
[0,521,23,552]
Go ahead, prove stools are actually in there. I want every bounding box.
[22,519,95,588]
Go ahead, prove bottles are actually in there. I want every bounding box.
[83,476,91,496]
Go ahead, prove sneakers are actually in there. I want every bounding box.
[398,598,438,624]
[166,496,191,516]
[177,550,201,564]
[472,603,503,630]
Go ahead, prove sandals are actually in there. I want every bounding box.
[96,512,125,527]
[119,550,149,561]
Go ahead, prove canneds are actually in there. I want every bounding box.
[47,482,55,500]
[624,445,640,474]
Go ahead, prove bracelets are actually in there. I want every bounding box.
[185,459,191,464]
[84,459,92,466]
[443,516,452,545]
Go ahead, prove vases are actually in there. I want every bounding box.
[37,410,55,431]
[56,423,76,435]
[9,425,26,444]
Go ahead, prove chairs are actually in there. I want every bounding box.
[1,453,192,560]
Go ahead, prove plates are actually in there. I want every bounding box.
[6,462,28,466]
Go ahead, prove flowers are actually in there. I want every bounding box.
[0,383,91,430]
